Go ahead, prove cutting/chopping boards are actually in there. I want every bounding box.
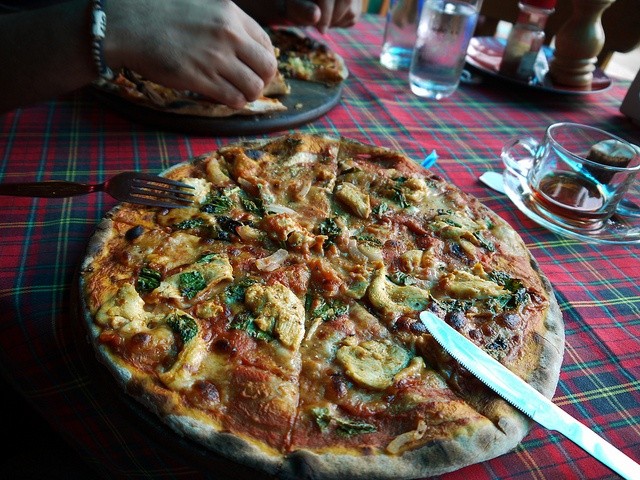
[85,76,343,137]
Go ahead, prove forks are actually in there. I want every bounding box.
[0,172,195,208]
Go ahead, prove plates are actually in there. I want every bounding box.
[464,36,613,111]
[503,158,640,245]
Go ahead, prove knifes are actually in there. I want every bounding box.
[419,309,640,480]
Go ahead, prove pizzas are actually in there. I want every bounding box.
[101,24,347,121]
[80,132,565,476]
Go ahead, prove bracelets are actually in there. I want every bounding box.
[91,0,124,83]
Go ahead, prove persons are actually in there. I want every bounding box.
[1,1,363,122]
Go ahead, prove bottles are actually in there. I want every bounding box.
[499,1,555,81]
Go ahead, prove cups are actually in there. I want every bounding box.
[501,122,640,231]
[408,0,483,99]
[379,0,425,71]
